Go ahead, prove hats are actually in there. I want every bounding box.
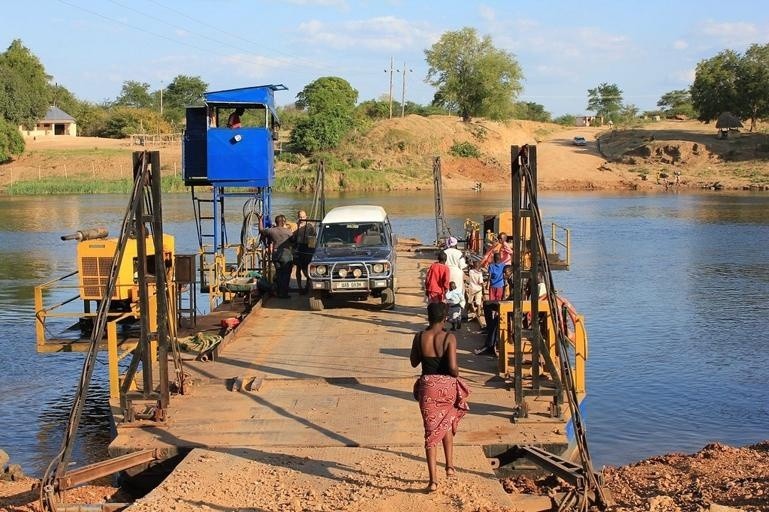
[447,236,457,247]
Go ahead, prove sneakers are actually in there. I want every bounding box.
[278,294,292,299]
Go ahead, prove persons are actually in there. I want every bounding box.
[228,106,245,129]
[409,300,472,494]
[290,209,317,298]
[424,216,547,357]
[253,209,296,301]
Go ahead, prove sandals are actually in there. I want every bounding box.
[446,466,455,476]
[428,482,437,491]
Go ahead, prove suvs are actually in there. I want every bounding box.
[574,136,586,145]
[293,203,400,312]
